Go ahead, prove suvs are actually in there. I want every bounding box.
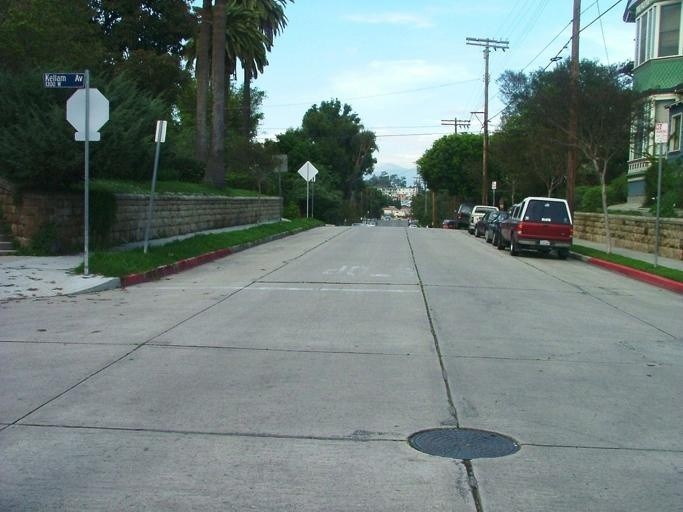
[454,202,474,230]
[497,196,575,260]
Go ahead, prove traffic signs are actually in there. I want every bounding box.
[43,72,85,89]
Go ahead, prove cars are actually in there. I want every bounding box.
[441,219,456,229]
[352,214,420,228]
[474,209,499,238]
[484,210,510,246]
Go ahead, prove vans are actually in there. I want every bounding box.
[468,205,499,235]
